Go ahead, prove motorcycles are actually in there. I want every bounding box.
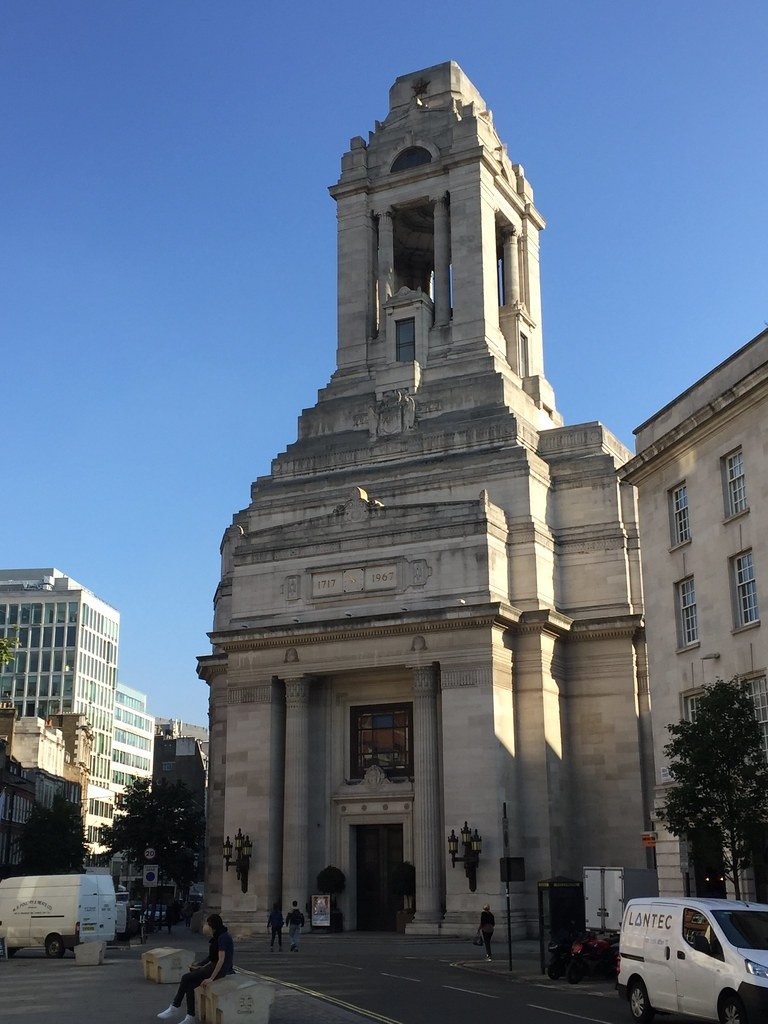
[545,930,610,984]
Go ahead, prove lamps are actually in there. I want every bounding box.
[446,821,482,892]
[223,826,253,894]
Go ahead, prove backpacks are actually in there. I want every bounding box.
[290,909,301,925]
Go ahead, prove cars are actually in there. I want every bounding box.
[144,901,166,921]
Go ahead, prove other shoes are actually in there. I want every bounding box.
[270,947,274,952]
[157,1005,180,1019]
[294,949,298,952]
[178,1014,196,1024]
[291,944,296,951]
[279,947,283,952]
[484,954,492,962]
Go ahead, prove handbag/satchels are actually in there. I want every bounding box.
[473,930,483,946]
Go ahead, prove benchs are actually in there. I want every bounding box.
[194,973,275,1024]
[73,941,108,965]
[141,946,195,984]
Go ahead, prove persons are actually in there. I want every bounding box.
[287,901,304,951]
[478,903,495,962]
[267,904,285,952]
[157,914,236,1024]
[165,898,199,934]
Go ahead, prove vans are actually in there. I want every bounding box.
[0,875,116,959]
[617,896,768,1024]
